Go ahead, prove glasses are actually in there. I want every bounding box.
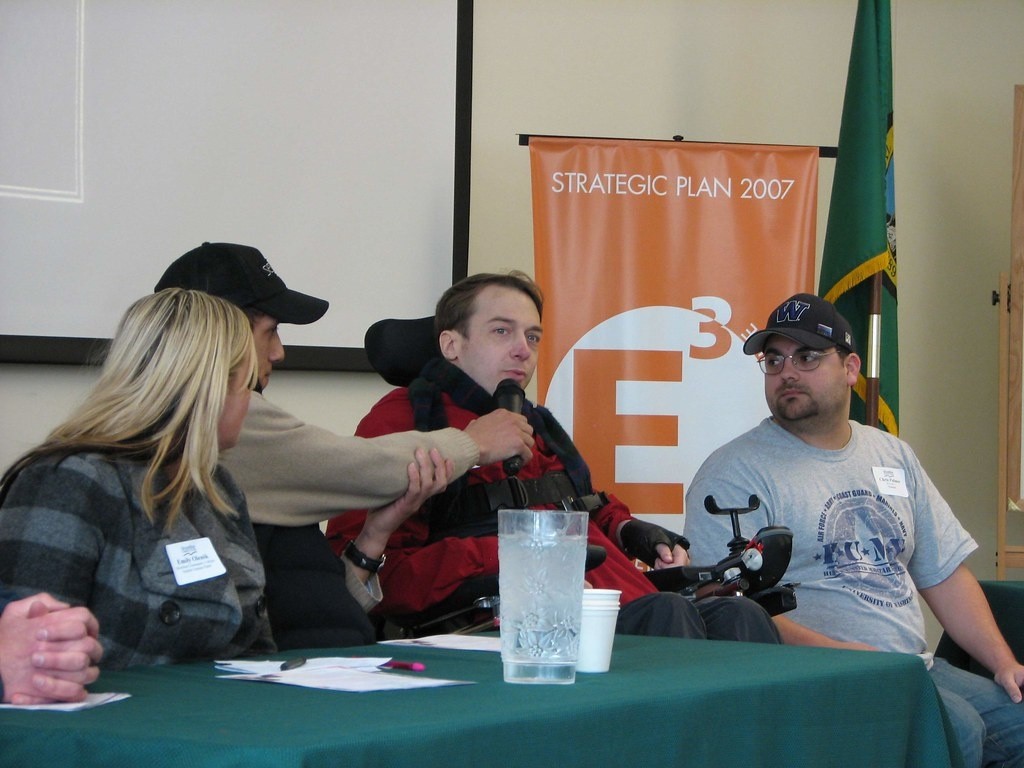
[757,350,840,375]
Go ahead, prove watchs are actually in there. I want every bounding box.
[343,539,387,574]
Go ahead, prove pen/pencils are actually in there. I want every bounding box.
[353,656,425,672]
[281,657,306,671]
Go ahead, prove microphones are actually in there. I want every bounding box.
[493,379,525,473]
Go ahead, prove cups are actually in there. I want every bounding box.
[575,588,622,673]
[498,509,591,685]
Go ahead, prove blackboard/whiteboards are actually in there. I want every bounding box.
[0,0,474,373]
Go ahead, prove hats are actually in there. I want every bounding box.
[153,242,329,325]
[743,293,856,355]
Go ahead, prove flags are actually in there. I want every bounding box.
[817,0,898,438]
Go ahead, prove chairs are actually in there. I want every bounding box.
[364,314,798,647]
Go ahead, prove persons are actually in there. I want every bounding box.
[153,243,535,652]
[0,591,103,706]
[683,293,1024,768]
[323,269,782,644]
[1,286,278,671]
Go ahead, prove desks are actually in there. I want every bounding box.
[0,632,964,768]
